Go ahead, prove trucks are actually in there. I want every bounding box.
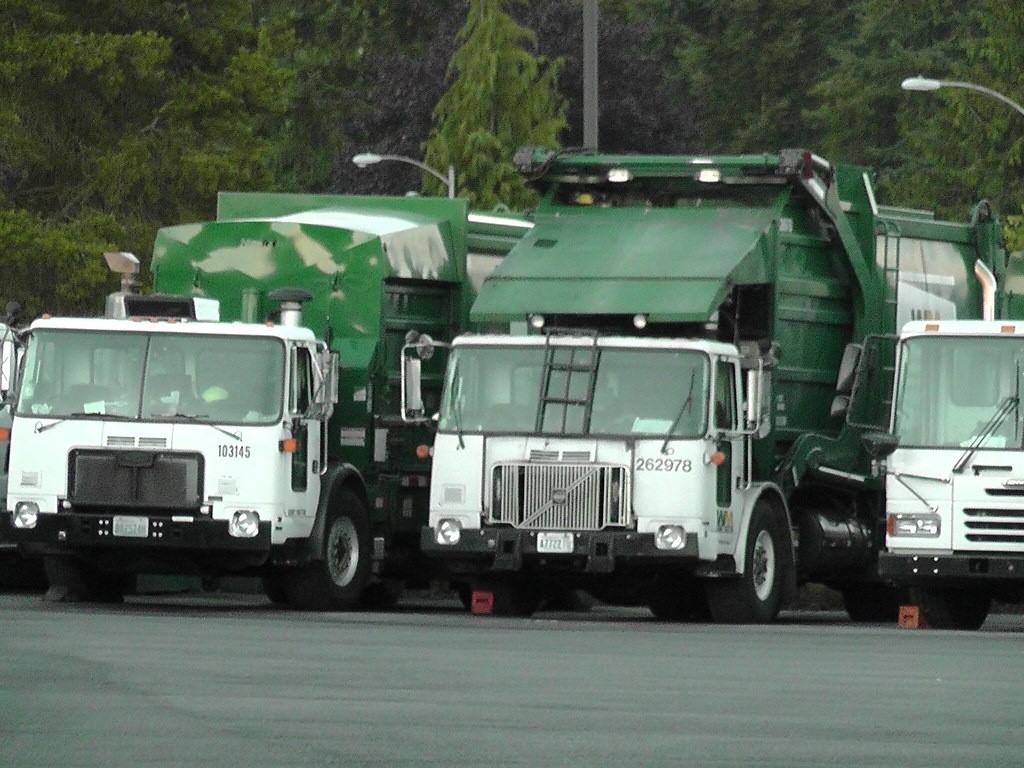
[0,144,1024,632]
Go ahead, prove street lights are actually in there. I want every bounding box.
[352,152,457,200]
